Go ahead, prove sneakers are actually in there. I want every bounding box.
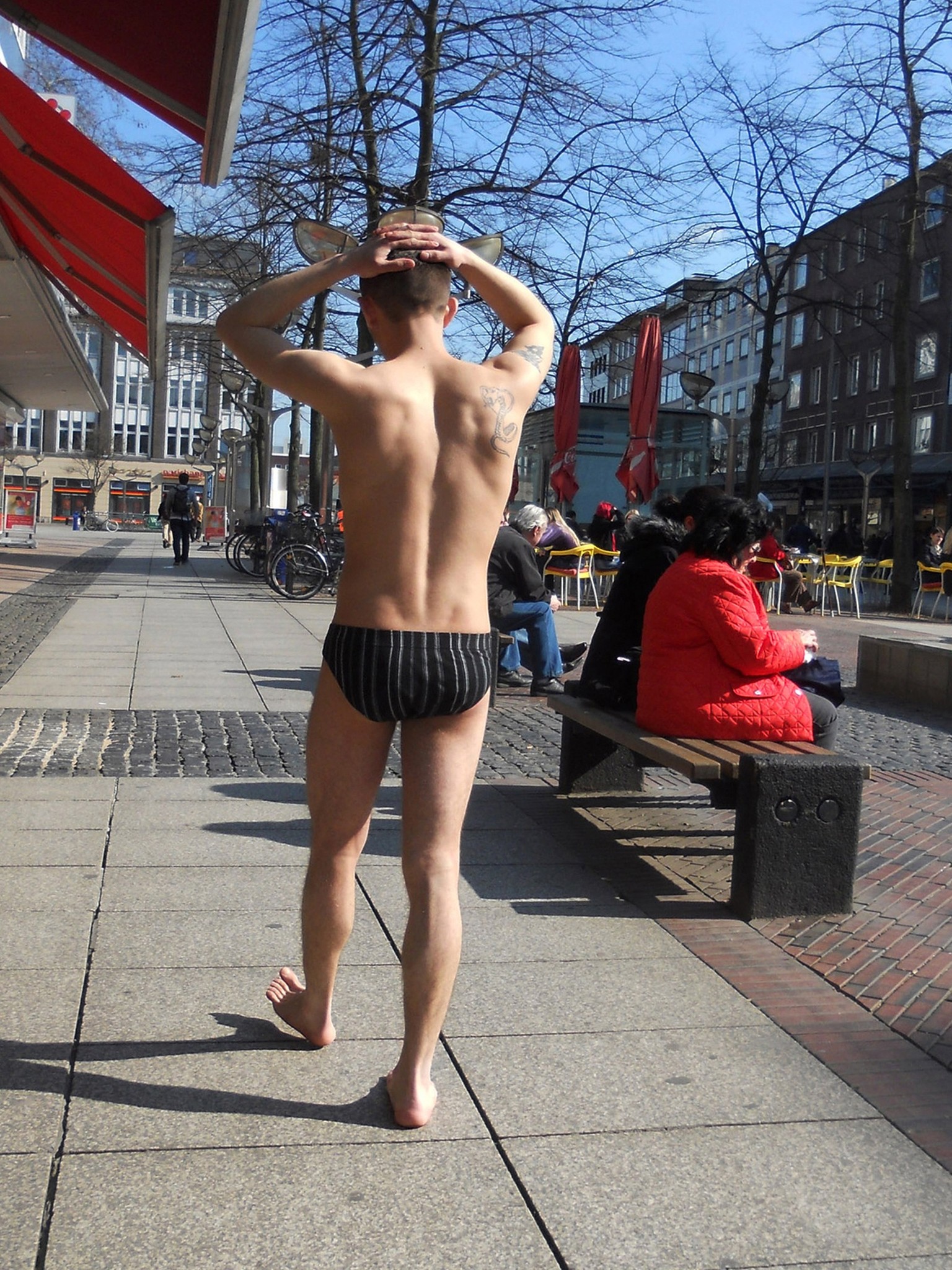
[530,676,564,696]
[496,670,533,688]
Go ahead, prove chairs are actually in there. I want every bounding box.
[534,542,952,622]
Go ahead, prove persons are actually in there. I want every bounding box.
[486,485,951,753]
[213,216,558,1132]
[157,472,204,566]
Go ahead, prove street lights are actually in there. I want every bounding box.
[108,466,139,528]
[680,370,791,497]
[844,447,901,554]
[185,414,256,539]
[4,450,44,491]
[221,369,306,513]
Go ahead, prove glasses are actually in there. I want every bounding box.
[751,545,761,557]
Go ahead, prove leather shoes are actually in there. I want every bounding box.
[559,642,587,664]
[561,657,584,674]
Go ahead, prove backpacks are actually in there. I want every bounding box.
[171,487,191,514]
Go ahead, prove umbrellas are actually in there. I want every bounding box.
[616,313,664,515]
[551,343,581,524]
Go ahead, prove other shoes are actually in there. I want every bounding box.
[804,600,822,611]
[182,559,187,564]
[168,544,170,547]
[775,603,792,614]
[174,561,179,565]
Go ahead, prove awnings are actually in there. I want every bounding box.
[1,0,263,190]
[1,61,176,381]
[0,225,110,414]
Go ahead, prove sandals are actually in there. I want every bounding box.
[163,541,168,548]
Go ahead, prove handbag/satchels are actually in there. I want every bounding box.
[780,656,845,708]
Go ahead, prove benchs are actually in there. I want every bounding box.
[547,694,872,922]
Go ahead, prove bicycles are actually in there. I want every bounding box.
[225,503,345,600]
[85,511,119,533]
